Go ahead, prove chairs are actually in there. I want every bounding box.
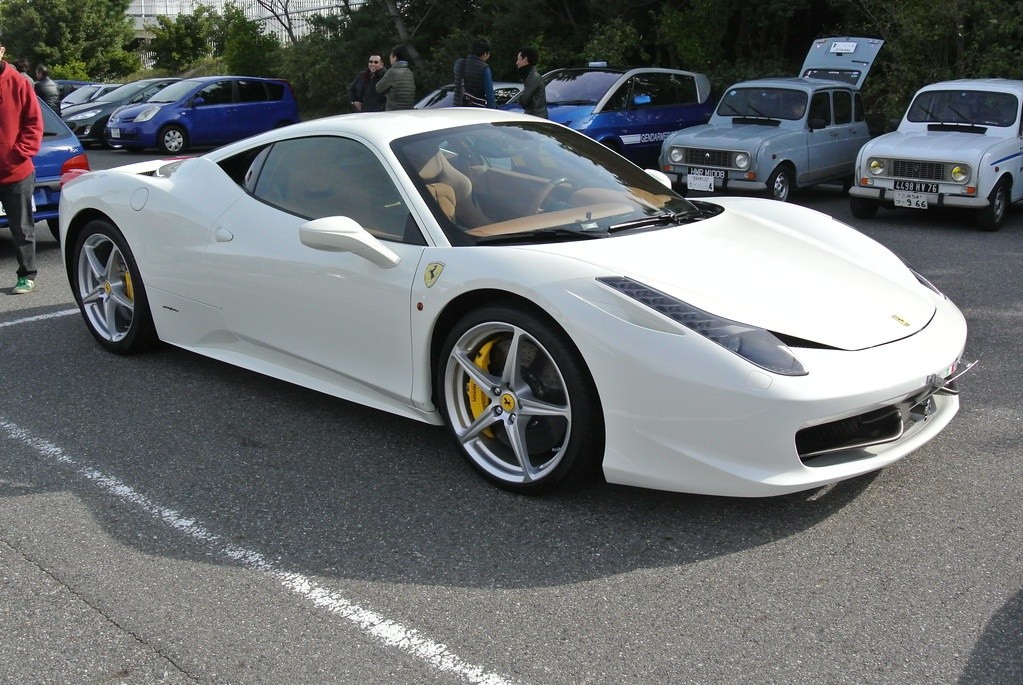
[260,146,396,236]
[397,141,494,239]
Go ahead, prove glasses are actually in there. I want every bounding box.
[369,60,380,64]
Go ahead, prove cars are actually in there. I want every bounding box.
[54,75,183,148]
[847,77,1023,231]
[411,82,520,107]
[0,79,91,247]
[655,35,894,200]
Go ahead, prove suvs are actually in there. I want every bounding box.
[501,64,717,158]
[104,74,299,156]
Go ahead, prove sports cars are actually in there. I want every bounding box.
[58,105,980,499]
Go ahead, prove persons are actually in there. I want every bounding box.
[791,100,805,116]
[0,30,44,293]
[516,47,549,120]
[376,45,415,111]
[452,39,497,109]
[34,65,61,118]
[13,58,35,87]
[349,54,387,113]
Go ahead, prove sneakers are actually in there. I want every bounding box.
[12,278,34,294]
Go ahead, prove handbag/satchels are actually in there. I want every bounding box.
[460,87,487,108]
